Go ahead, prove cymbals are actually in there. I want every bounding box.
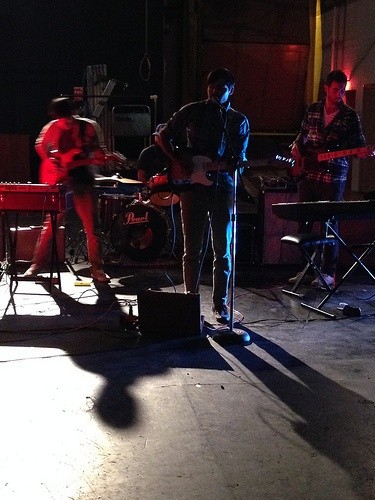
[94,174,143,184]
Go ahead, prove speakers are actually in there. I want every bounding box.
[337,190,375,267]
[1,134,32,182]
[137,288,201,339]
[7,225,66,263]
[259,189,306,265]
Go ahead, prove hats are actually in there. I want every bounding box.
[152,123,168,136]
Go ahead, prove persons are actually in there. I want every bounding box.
[289,70,375,288]
[23,96,123,282]
[137,124,189,263]
[153,69,250,323]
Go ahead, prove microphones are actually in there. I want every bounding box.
[209,95,227,113]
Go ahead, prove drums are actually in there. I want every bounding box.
[110,201,171,262]
[146,171,179,205]
[98,193,139,229]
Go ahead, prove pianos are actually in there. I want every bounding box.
[271,199,375,318]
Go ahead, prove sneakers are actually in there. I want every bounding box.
[289,272,311,282]
[23,266,42,277]
[311,273,334,285]
[91,268,111,281]
[213,306,229,321]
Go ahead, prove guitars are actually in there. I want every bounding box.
[292,145,375,176]
[39,148,127,187]
[168,154,295,196]
